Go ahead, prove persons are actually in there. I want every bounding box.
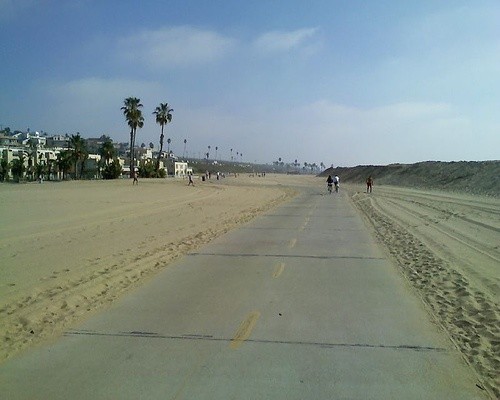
[205,170,220,180]
[326,175,333,192]
[366,176,374,194]
[133,171,139,184]
[38,173,43,184]
[333,175,339,186]
[188,173,195,186]
